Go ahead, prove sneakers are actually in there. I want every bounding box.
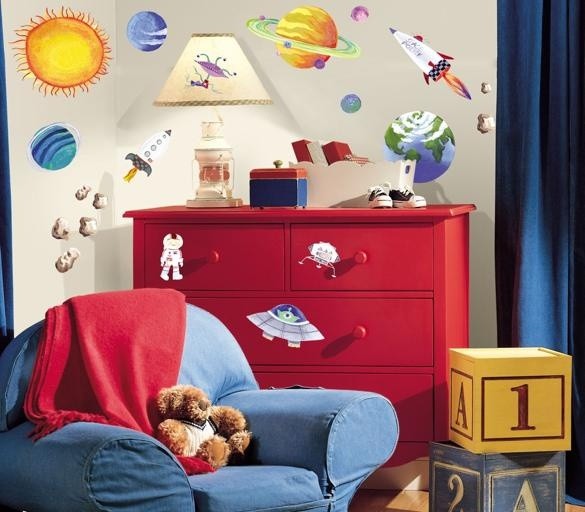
[367,185,393,209]
[389,184,427,208]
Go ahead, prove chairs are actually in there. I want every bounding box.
[0,289,402,511]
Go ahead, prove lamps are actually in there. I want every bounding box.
[153,33,279,206]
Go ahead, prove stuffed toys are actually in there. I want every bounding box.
[150,381,251,469]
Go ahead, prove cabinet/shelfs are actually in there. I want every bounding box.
[122,203,477,443]
